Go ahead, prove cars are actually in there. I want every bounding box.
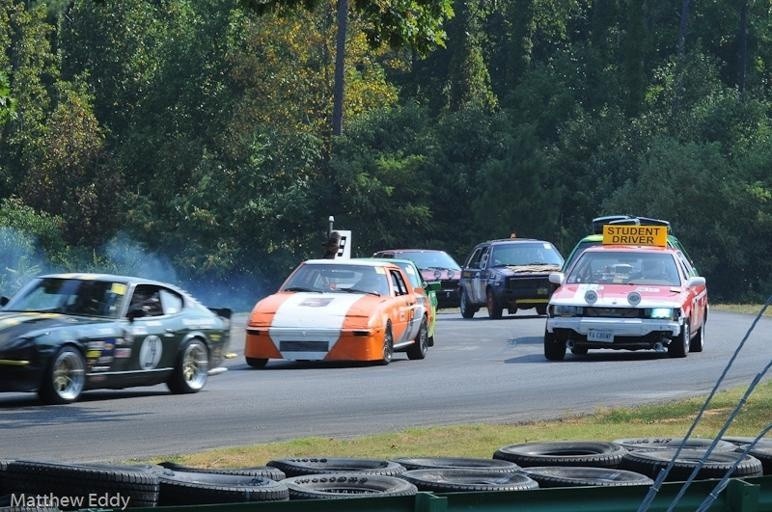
[0,273,233,403]
[371,249,463,311]
[355,258,442,347]
[543,225,708,360]
[243,257,433,368]
[560,215,701,276]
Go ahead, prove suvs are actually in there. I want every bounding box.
[459,237,566,319]
